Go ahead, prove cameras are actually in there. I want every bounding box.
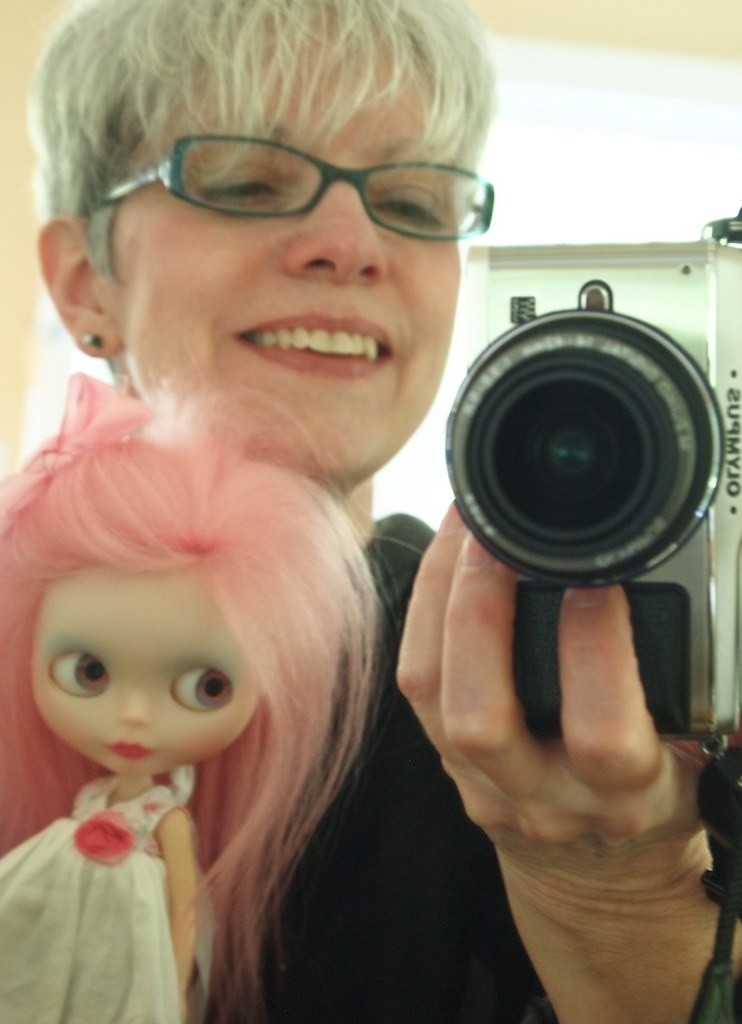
[440,242,742,737]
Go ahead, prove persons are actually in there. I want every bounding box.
[26,0,742,1024]
[0,376,384,1024]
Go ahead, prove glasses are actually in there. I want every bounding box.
[74,136,494,239]
